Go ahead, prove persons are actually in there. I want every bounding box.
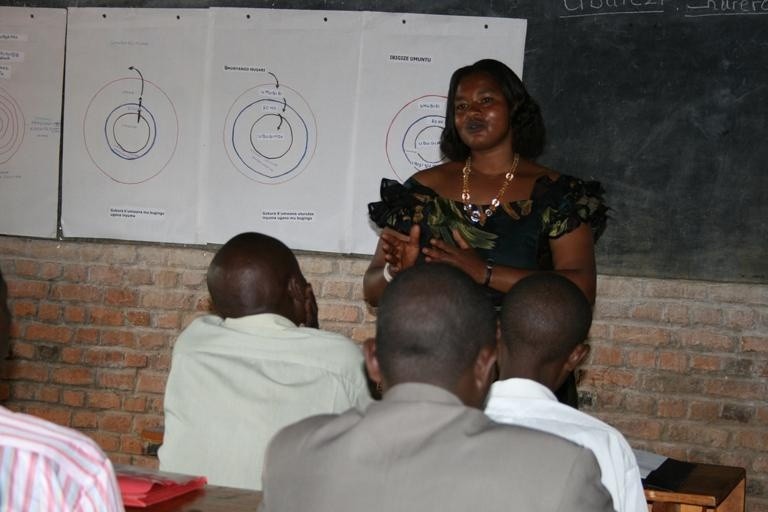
[157,232,375,491]
[257,261,619,512]
[482,273,649,512]
[362,59,608,411]
[0,405,126,512]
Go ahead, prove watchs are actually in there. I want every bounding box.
[483,258,496,287]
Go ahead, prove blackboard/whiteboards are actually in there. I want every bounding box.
[0,0,768,286]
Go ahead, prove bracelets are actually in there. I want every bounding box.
[383,263,394,283]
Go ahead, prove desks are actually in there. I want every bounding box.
[641,459,747,512]
[124,486,264,512]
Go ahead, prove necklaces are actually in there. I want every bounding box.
[462,152,520,227]
